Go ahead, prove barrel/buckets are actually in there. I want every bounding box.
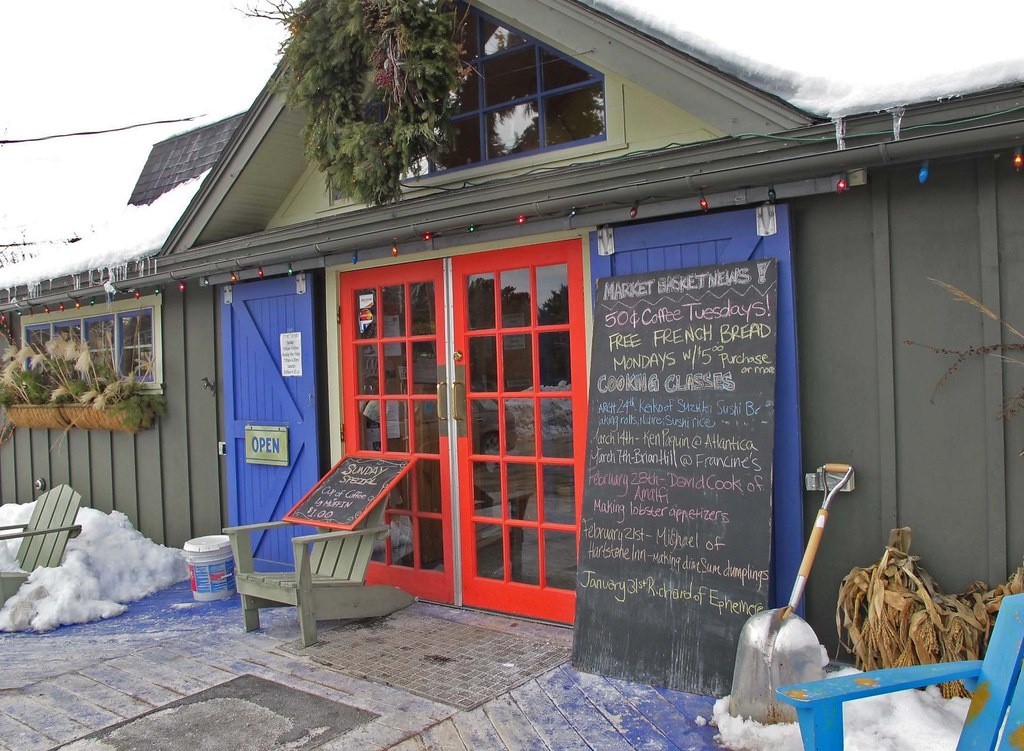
[184,535,236,601]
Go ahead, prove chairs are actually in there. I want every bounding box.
[776,593,1024,750]
[0,484,87,612]
[221,491,418,649]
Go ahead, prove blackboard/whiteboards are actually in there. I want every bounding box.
[283,452,419,531]
[573,258,778,698]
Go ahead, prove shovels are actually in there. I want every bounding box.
[730,461,856,726]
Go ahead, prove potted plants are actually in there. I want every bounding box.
[0,337,169,433]
